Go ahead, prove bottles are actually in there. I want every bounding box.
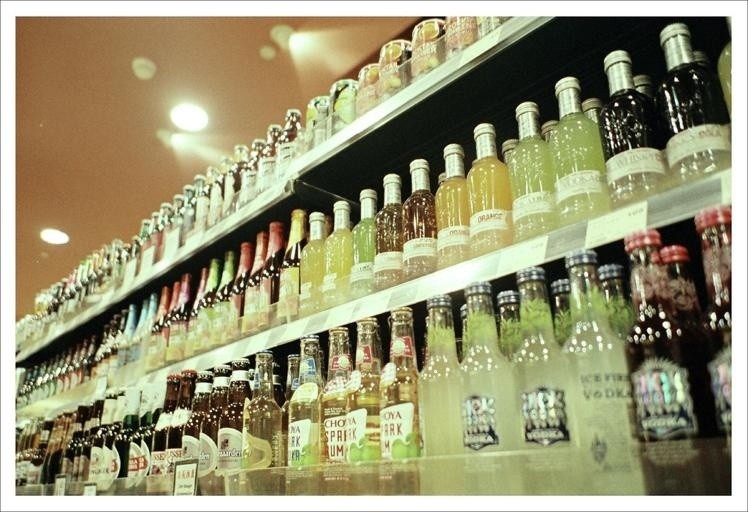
[13,182,194,412]
[16,358,252,493]
[372,23,733,291]
[188,108,439,351]
[418,226,729,494]
[244,307,418,496]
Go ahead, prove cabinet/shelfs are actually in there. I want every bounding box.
[17,16,731,486]
